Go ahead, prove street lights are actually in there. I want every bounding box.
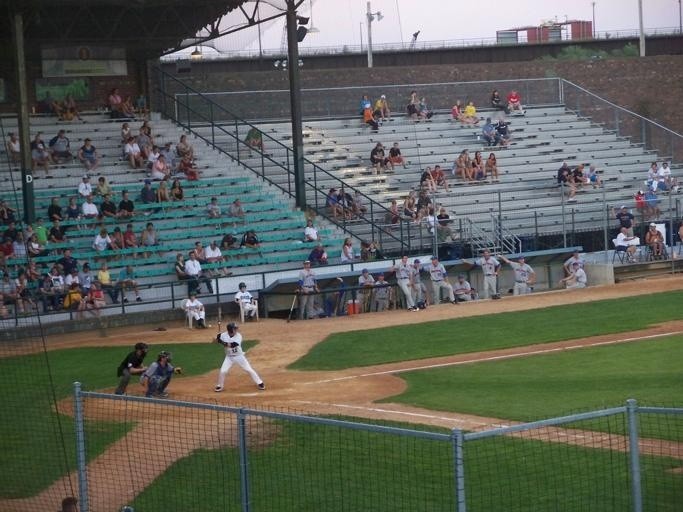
[364,1,383,68]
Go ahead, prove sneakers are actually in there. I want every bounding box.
[258,383,265,390]
[215,387,221,391]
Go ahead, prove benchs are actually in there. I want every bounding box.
[177,103,683,251]
[0,107,362,331]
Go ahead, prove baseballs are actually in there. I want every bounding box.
[208,325,212,327]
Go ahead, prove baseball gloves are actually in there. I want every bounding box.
[172,366,182,375]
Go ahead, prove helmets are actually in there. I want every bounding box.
[160,351,171,363]
[226,323,238,329]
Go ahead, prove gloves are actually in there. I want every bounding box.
[217,333,223,344]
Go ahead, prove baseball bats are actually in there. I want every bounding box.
[216,307,221,343]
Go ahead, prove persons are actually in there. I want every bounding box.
[114,342,149,395]
[0,88,682,332]
[139,351,181,398]
[212,322,266,393]
[61,496,78,512]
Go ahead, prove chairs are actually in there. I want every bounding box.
[610,231,683,264]
